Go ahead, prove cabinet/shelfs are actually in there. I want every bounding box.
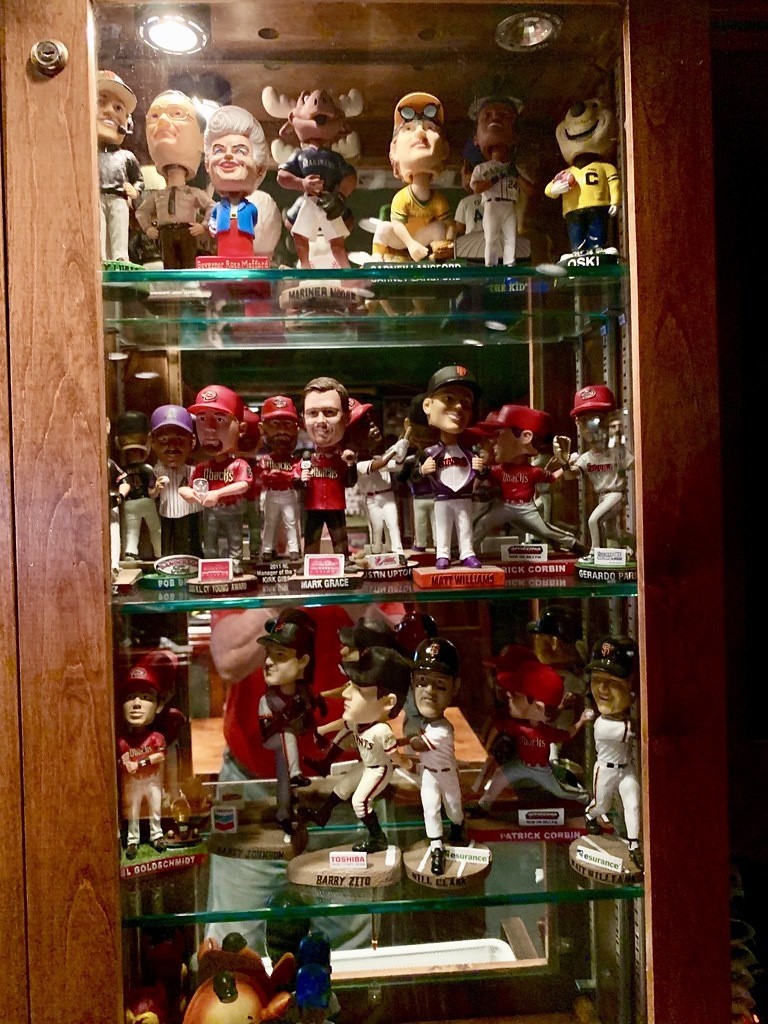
[0,0,732,1022]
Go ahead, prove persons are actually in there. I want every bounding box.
[91,71,638,1024]
[204,489,406,1014]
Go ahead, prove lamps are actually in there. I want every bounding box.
[134,1,213,56]
[494,2,565,53]
